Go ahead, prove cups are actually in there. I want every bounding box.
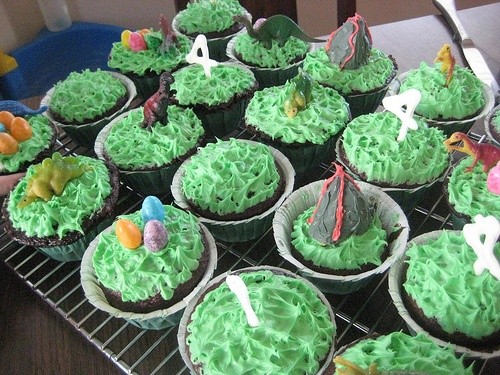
[37,0,72,32]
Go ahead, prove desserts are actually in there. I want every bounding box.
[0,0,500,375]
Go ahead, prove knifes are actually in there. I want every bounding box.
[432,0,500,105]
